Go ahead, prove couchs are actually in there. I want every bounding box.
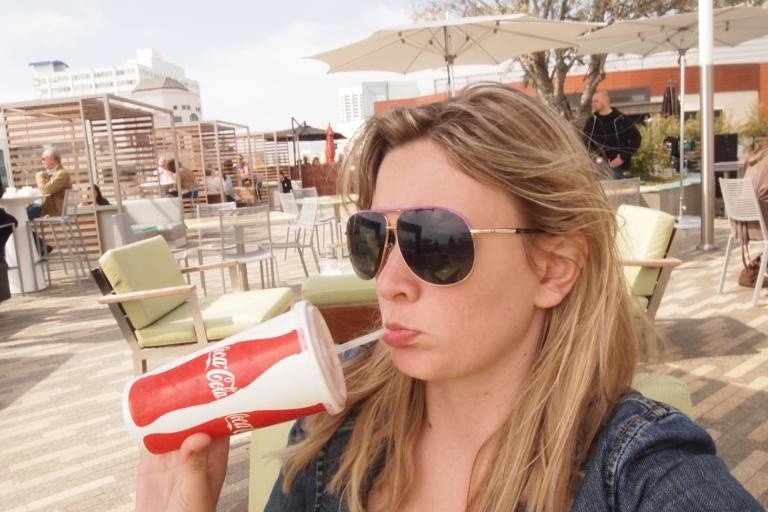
[108,197,187,249]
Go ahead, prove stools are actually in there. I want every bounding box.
[300,273,382,345]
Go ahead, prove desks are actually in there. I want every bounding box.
[138,182,174,197]
[286,193,360,259]
[714,158,745,178]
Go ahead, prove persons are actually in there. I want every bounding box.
[79,182,111,209]
[279,170,293,194]
[158,157,177,193]
[312,156,320,165]
[167,157,200,198]
[733,140,767,288]
[582,89,642,179]
[26,146,72,262]
[201,154,254,204]
[128,81,768,510]
[303,157,311,165]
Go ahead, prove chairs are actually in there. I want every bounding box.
[597,176,640,211]
[170,174,342,296]
[88,234,298,375]
[0,189,91,296]
[714,177,765,311]
[596,202,684,362]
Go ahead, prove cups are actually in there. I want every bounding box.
[123,301,349,456]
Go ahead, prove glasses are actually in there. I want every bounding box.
[346,208,548,289]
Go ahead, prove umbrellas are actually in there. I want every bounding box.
[326,122,335,164]
[576,3,767,216]
[303,8,608,102]
[658,78,681,160]
